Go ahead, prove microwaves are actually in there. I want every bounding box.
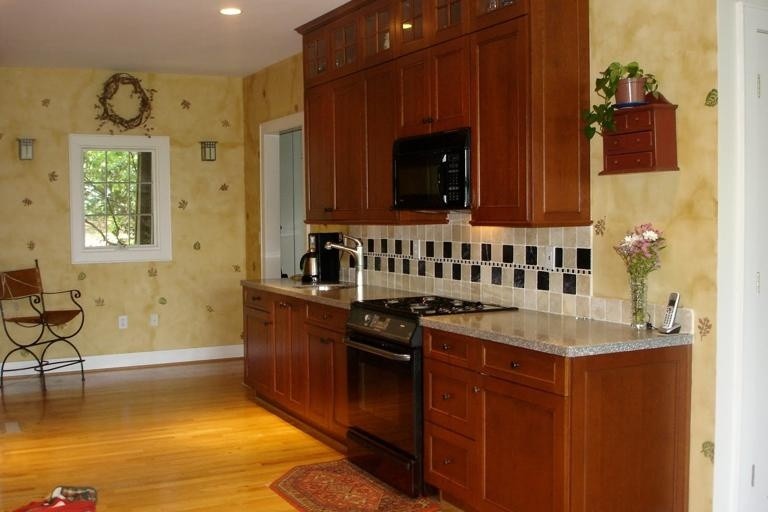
[392,126,472,215]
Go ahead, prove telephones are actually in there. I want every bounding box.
[659,292,681,335]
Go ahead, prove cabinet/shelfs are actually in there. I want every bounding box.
[469,1,590,230]
[599,104,681,175]
[395,0,469,137]
[243,286,349,456]
[294,0,368,226]
[423,326,691,512]
[360,0,449,227]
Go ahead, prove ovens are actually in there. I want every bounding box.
[345,310,424,499]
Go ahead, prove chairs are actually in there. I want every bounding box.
[0,260,84,392]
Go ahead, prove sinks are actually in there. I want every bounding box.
[292,282,356,293]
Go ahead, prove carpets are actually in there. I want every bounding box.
[270,458,442,511]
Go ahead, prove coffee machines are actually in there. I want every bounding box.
[300,232,344,284]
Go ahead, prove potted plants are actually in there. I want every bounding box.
[581,61,658,139]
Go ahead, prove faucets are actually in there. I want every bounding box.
[355,286,364,303]
[322,235,364,287]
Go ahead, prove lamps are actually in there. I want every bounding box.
[201,141,217,161]
[17,138,35,160]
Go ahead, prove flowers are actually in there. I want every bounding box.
[612,224,667,324]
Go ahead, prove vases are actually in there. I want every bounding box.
[628,277,648,331]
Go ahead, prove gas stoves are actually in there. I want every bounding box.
[354,292,518,317]
